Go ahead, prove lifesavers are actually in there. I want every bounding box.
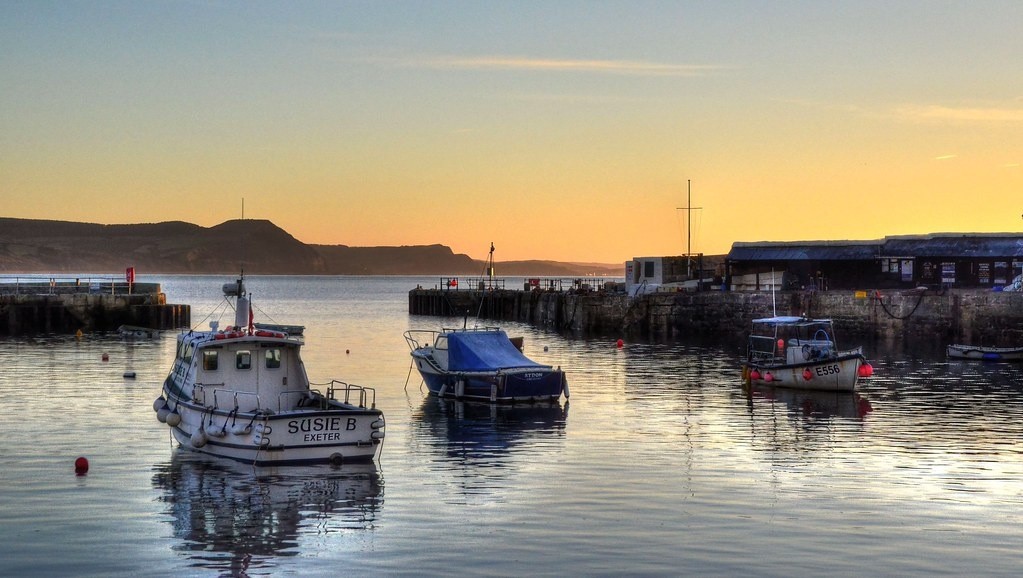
[214,331,246,340]
[255,329,286,338]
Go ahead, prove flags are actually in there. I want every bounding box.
[249,295,254,334]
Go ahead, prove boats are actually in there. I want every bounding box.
[118,324,160,339]
[403,309,565,403]
[153,446,381,578]
[161,199,385,464]
[417,392,566,454]
[946,344,1023,361]
[744,266,863,392]
[749,386,866,428]
